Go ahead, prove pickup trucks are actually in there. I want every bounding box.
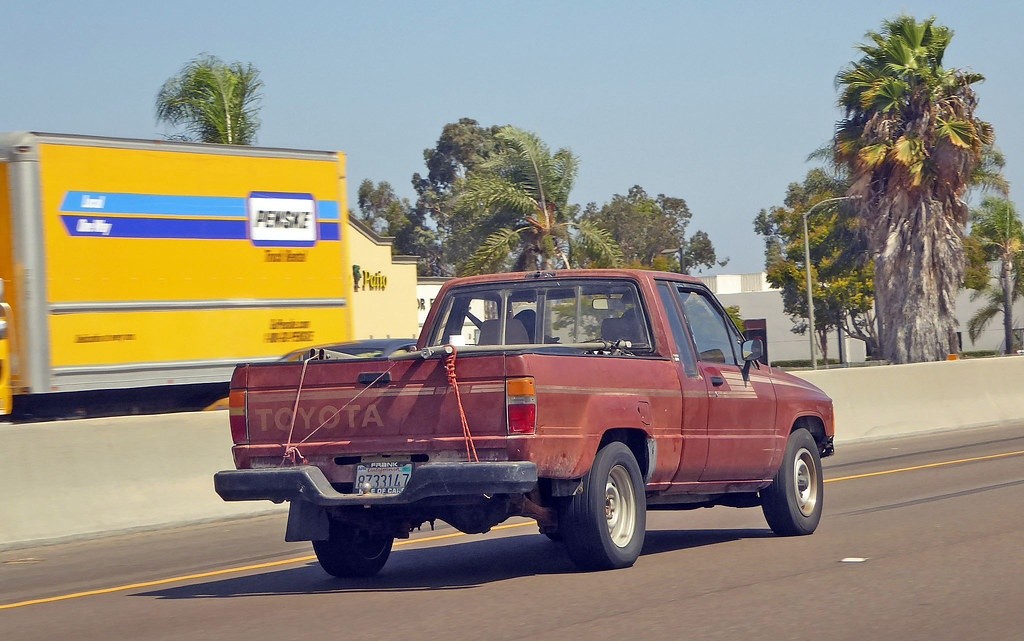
[213,268,835,585]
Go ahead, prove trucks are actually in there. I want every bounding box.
[3,132,358,423]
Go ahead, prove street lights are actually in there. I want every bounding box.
[802,193,864,369]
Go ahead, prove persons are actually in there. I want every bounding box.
[510,309,563,347]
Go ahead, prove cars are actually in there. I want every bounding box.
[266,339,421,362]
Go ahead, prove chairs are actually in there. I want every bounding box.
[600,317,646,347]
[477,318,529,345]
